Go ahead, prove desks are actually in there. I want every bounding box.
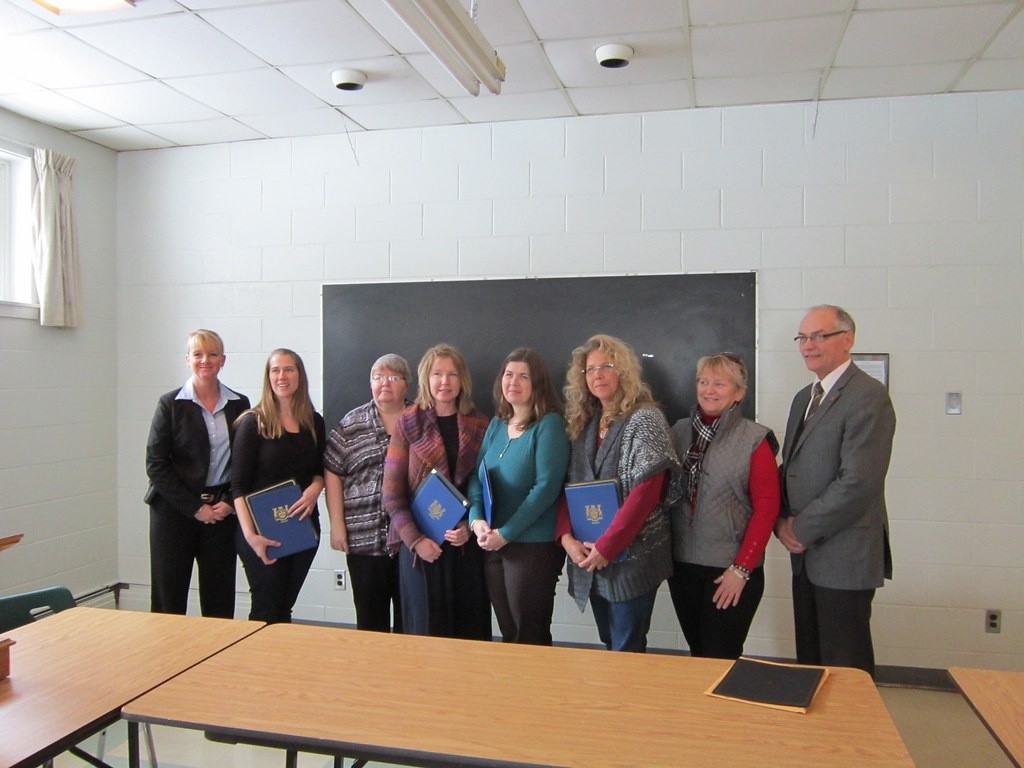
[0,607,266,768]
[121,622,916,768]
[945,663,1024,768]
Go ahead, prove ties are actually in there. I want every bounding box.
[803,381,824,430]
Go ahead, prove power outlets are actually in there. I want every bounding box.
[985,609,1001,633]
[334,570,346,590]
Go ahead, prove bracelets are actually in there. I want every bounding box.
[734,564,750,573]
[196,507,202,514]
[729,566,750,580]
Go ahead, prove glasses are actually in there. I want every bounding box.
[581,362,617,376]
[370,375,406,383]
[794,330,848,344]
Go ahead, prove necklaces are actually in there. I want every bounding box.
[600,418,606,438]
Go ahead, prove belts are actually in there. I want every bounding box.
[200,493,220,503]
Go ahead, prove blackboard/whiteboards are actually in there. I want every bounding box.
[322,272,757,437]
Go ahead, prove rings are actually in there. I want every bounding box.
[721,575,723,578]
[205,522,208,524]
[331,544,336,546]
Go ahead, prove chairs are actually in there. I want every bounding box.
[0,586,77,633]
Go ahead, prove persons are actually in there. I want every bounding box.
[669,352,779,660]
[466,346,571,646]
[382,342,493,641]
[323,353,416,634]
[229,349,327,622]
[772,306,897,678]
[556,333,682,653]
[143,329,250,619]
